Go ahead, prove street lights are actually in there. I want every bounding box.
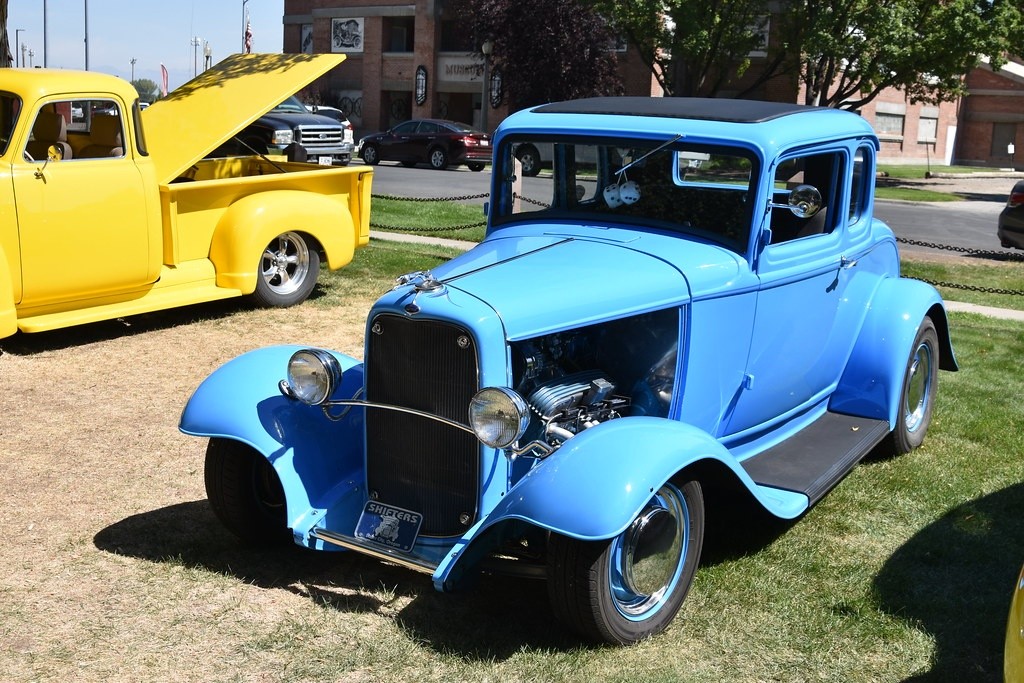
[129,58,136,83]
[191,36,201,78]
[28,48,35,68]
[15,28,26,68]
[479,37,494,132]
[21,43,28,69]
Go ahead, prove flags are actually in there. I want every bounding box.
[245,5,253,54]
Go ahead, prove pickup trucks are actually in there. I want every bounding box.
[1,53,375,343]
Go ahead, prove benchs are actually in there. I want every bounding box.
[583,173,827,243]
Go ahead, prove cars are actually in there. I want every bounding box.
[70,102,149,118]
[998,179,1024,251]
[358,118,493,171]
[511,140,711,181]
[177,98,959,651]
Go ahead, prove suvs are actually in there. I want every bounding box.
[278,104,355,149]
[203,95,357,165]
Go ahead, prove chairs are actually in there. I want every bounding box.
[26,111,74,161]
[78,112,124,158]
